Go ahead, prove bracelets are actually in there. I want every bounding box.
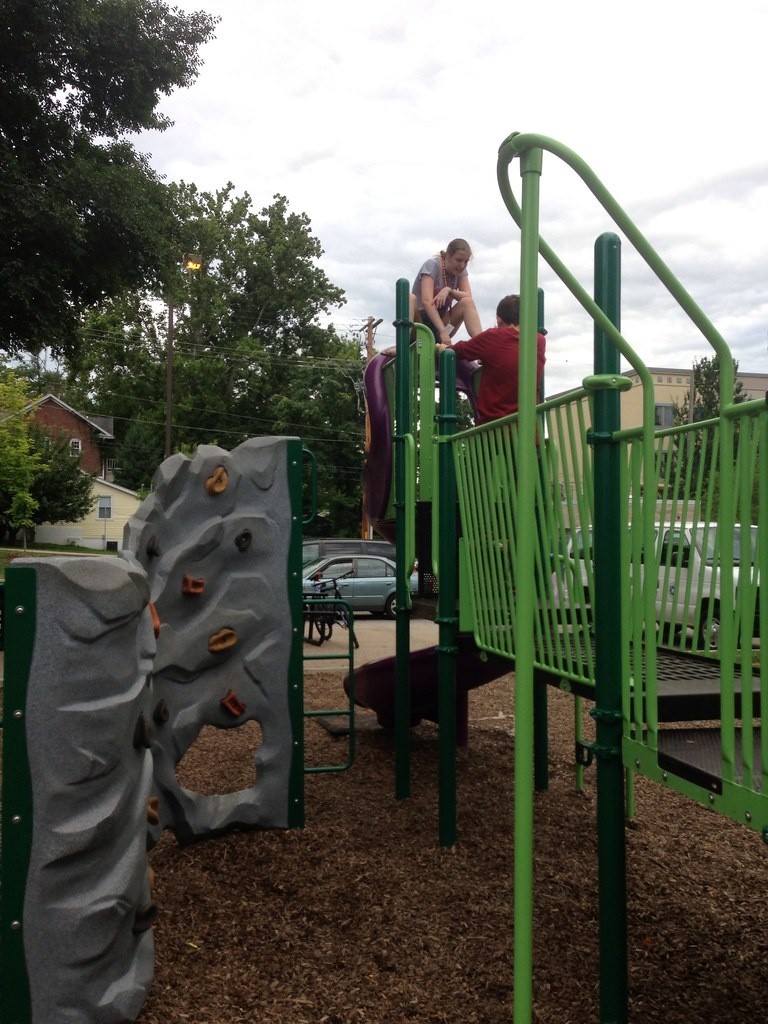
[449,287,454,295]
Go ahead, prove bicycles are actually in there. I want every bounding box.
[310,570,360,648]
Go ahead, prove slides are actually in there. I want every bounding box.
[343,346,515,730]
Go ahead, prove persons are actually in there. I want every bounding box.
[433,295,546,548]
[386,238,484,365]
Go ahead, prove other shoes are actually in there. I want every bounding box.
[381,346,396,356]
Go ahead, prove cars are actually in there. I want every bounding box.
[302,554,434,620]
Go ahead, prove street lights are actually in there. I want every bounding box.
[165,253,202,459]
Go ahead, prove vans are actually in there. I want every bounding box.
[302,538,418,571]
[564,522,768,650]
